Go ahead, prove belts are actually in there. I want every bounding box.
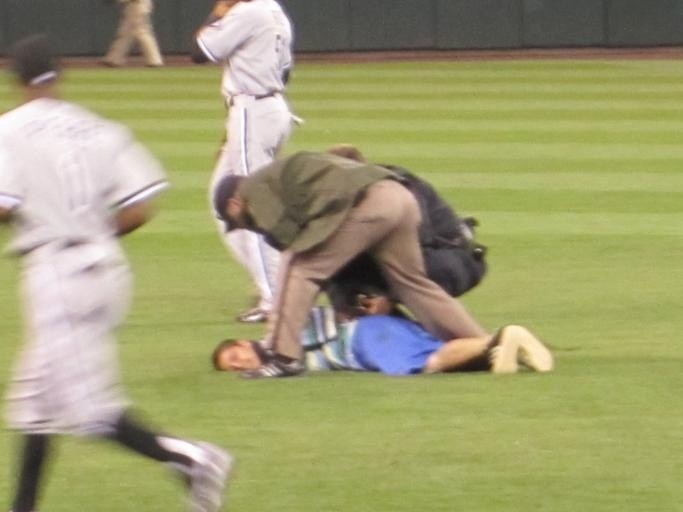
[224,91,275,109]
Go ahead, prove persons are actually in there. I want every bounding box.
[96,0,166,68]
[209,293,558,378]
[315,140,490,327]
[0,32,240,512]
[187,1,305,324]
[212,141,495,384]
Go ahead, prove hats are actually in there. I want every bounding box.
[13,36,59,91]
[212,175,247,234]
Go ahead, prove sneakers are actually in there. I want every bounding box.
[509,324,553,374]
[482,325,522,374]
[238,305,274,324]
[240,357,305,379]
